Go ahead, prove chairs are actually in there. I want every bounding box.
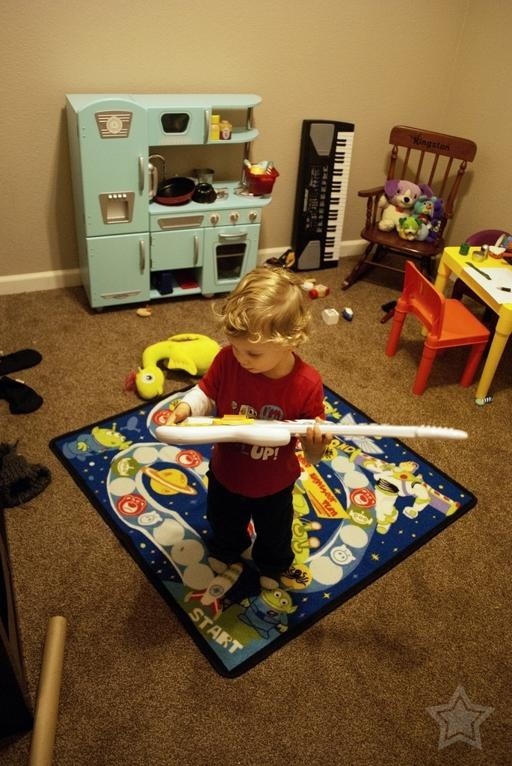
[385,259,490,395]
[454,230,512,322]
[339,125,477,290]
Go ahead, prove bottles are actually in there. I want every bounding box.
[211,115,232,140]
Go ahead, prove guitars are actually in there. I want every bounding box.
[156,414,468,447]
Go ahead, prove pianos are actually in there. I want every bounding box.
[289,118,355,272]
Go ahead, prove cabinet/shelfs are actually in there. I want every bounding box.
[65,91,273,313]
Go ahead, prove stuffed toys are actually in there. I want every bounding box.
[124,333,222,399]
[392,194,440,242]
[429,198,444,243]
[300,277,330,300]
[374,178,434,232]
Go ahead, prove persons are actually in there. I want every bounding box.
[162,265,335,586]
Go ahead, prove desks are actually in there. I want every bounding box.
[420,244,512,400]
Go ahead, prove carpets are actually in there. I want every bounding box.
[49,384,478,679]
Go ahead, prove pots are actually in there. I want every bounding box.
[153,178,194,204]
